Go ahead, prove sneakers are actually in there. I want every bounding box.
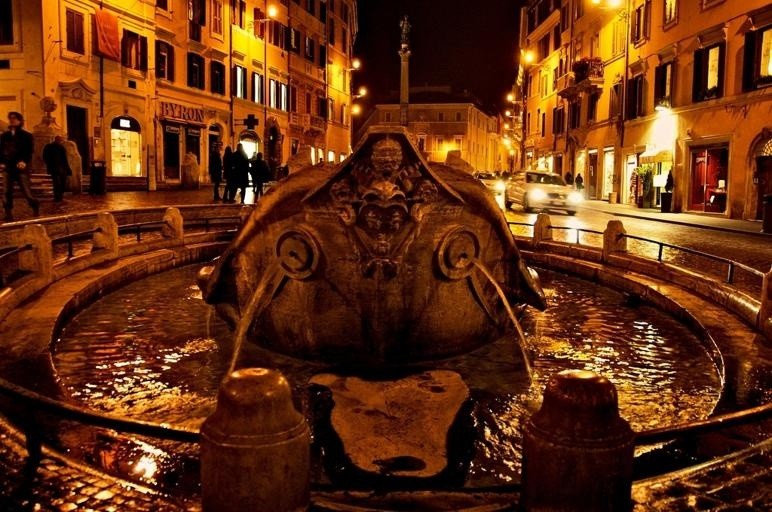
[214,197,245,204]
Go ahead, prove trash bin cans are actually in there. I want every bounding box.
[760,194,772,232]
[89,161,106,195]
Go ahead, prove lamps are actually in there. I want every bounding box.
[654,94,671,111]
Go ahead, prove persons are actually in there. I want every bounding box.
[565,172,574,185]
[43,137,72,202]
[0,112,40,220]
[575,174,584,190]
[209,143,269,203]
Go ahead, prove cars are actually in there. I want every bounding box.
[473,171,504,196]
[504,170,583,215]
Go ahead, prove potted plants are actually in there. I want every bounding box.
[657,170,677,212]
